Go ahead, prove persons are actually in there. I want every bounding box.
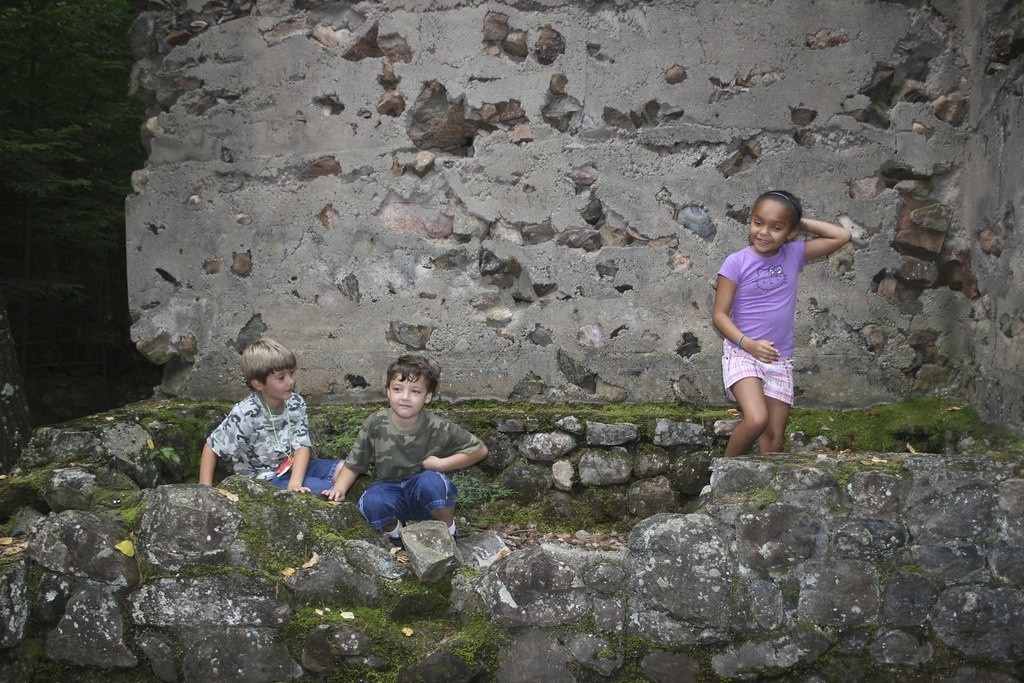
[712,190,852,457]
[198,339,346,493]
[322,354,488,539]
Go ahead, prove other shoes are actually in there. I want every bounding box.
[389,521,408,550]
[451,531,457,542]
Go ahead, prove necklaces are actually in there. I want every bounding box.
[262,398,295,473]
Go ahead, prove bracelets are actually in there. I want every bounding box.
[737,334,747,349]
